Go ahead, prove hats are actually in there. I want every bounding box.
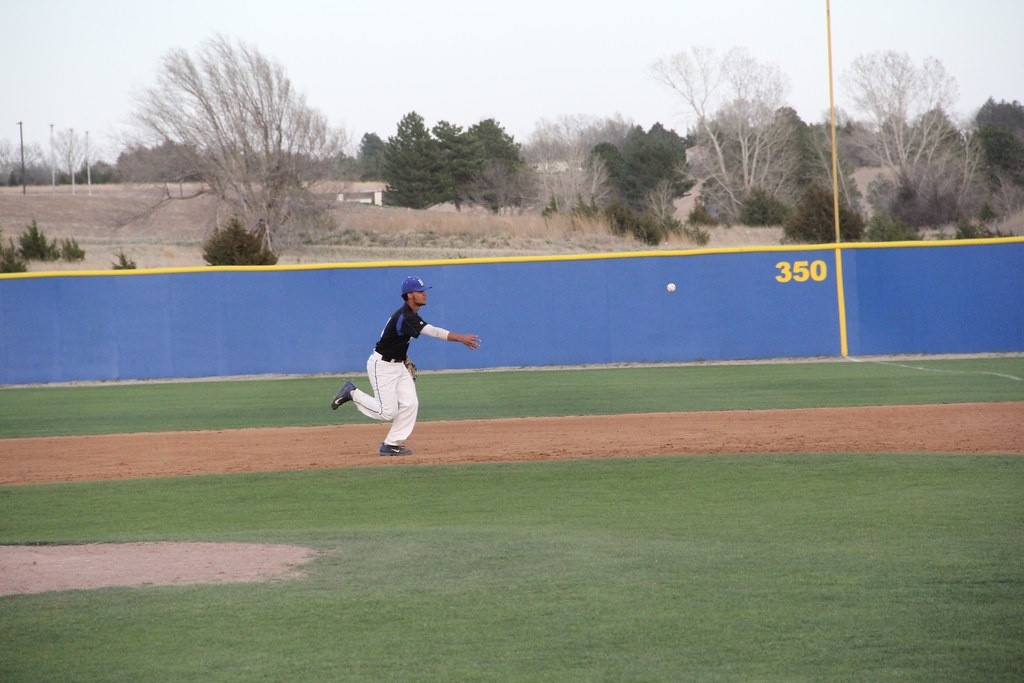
[401,276,432,295]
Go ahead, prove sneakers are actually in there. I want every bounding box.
[331,380,355,410]
[379,444,413,455]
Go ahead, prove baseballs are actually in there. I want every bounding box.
[666,282,676,292]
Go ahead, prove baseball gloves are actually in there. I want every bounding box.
[404,357,418,382]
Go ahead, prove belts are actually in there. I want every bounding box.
[382,355,403,363]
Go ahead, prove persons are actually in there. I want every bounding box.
[331,277,481,456]
